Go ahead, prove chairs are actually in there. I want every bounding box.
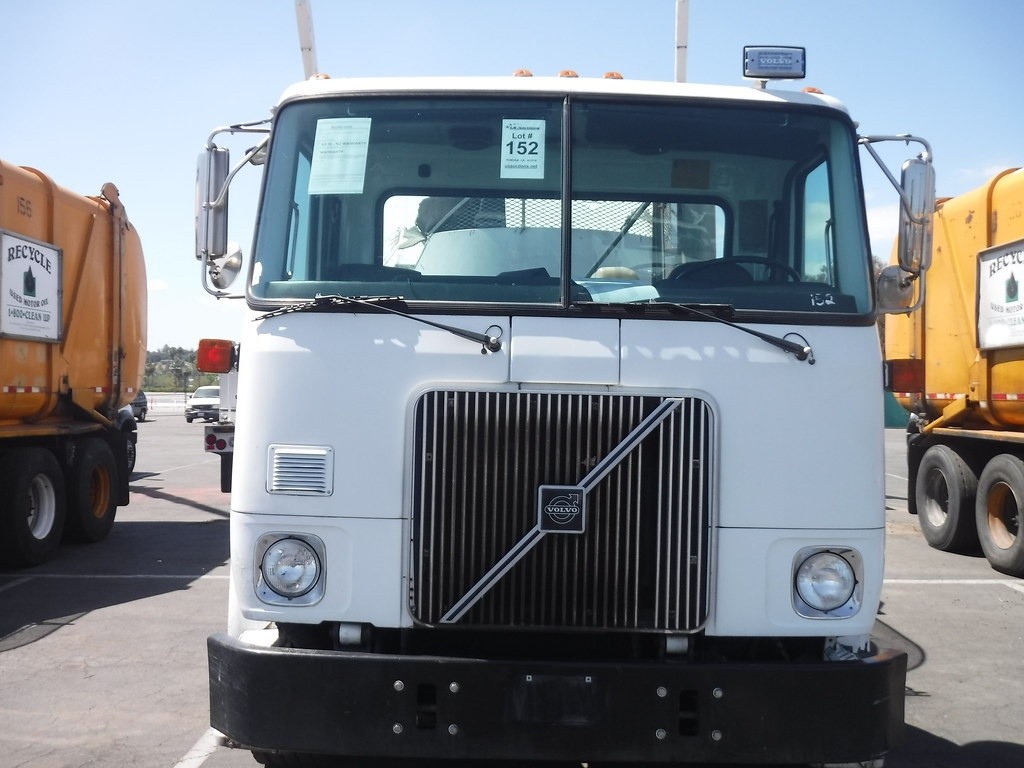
[678,263,753,281]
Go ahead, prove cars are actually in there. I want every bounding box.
[131,390,148,422]
[184,385,221,423]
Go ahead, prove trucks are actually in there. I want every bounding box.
[192,46,936,768]
